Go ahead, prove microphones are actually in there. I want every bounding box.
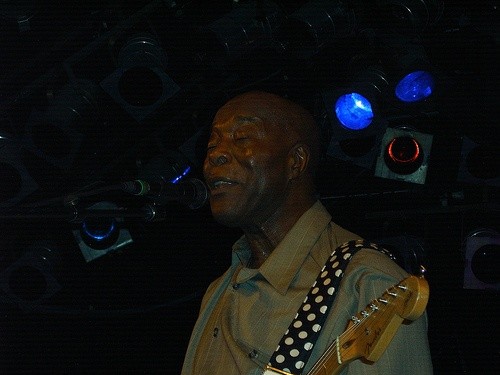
[69,200,167,224]
[67,178,208,212]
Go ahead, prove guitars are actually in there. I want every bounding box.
[306,276,429,375]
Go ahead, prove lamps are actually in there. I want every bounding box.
[31,81,105,160]
[392,57,435,103]
[371,123,433,186]
[1,238,66,315]
[116,31,175,109]
[335,68,392,133]
[68,195,131,265]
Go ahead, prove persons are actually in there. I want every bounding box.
[183,90,434,375]
